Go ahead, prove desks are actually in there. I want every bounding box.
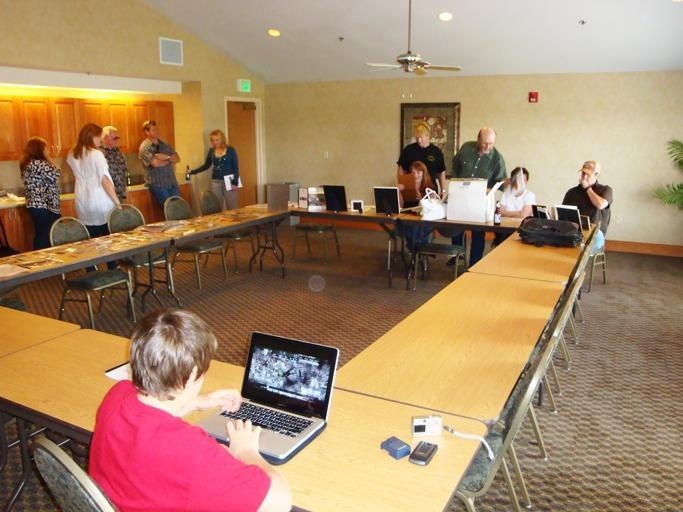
[1,307,487,510]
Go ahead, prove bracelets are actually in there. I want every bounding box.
[584,186,592,192]
[167,155,171,160]
[441,189,446,193]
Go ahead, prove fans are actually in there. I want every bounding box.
[361,0,461,82]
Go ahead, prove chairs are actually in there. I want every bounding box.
[584,208,612,293]
[289,196,604,511]
[1,192,292,329]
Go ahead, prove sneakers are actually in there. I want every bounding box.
[446,254,466,266]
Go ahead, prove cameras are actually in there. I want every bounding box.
[411,414,443,436]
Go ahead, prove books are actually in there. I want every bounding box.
[142,219,196,238]
[298,187,326,212]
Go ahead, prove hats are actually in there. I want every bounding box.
[577,160,601,176]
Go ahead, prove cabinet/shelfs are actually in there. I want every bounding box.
[71,95,128,156]
[126,98,173,155]
[18,93,82,164]
[0,95,23,161]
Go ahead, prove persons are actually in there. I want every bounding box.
[139,118,182,206]
[446,127,507,268]
[19,137,63,249]
[494,167,537,218]
[100,126,129,205]
[397,121,446,202]
[185,129,239,210]
[66,123,123,271]
[88,309,293,512]
[562,160,613,256]
[398,161,436,259]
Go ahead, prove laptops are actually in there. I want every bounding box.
[323,185,351,211]
[373,187,402,214]
[192,330,340,467]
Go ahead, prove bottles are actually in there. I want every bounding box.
[493,201,501,225]
[127,173,131,186]
[185,165,190,182]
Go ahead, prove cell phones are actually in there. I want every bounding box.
[408,440,438,466]
